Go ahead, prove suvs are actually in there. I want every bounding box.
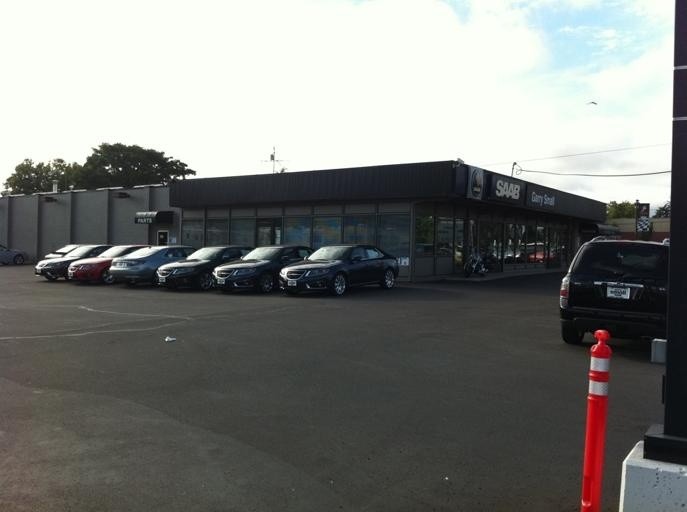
[559,236,670,344]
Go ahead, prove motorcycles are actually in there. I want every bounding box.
[464,246,490,277]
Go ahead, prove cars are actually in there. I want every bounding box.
[67,245,151,285]
[210,246,317,294]
[529,251,556,264]
[0,244,28,265]
[34,244,112,280]
[111,245,198,285]
[154,246,254,291]
[455,246,463,265]
[279,244,399,297]
